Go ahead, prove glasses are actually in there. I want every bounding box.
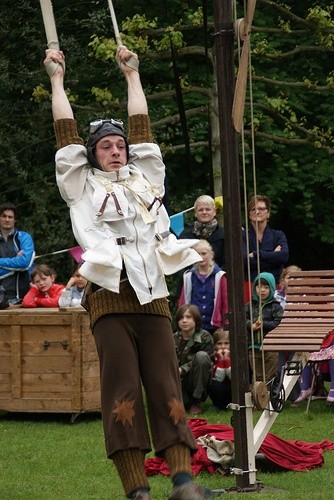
[89,119,124,133]
[250,207,269,213]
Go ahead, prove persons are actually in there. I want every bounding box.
[59,263,88,308]
[177,194,228,278]
[241,194,292,284]
[243,272,284,391]
[176,241,228,330]
[0,202,35,308]
[273,264,314,368]
[22,263,64,307]
[293,329,334,403]
[170,304,214,415]
[207,326,237,410]
[42,45,205,500]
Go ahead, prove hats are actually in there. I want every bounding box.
[87,123,129,170]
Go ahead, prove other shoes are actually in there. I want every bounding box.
[169,482,212,500]
[326,389,334,402]
[189,398,203,415]
[131,489,150,500]
[293,388,313,403]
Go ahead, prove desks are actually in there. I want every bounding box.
[0,308,101,421]
